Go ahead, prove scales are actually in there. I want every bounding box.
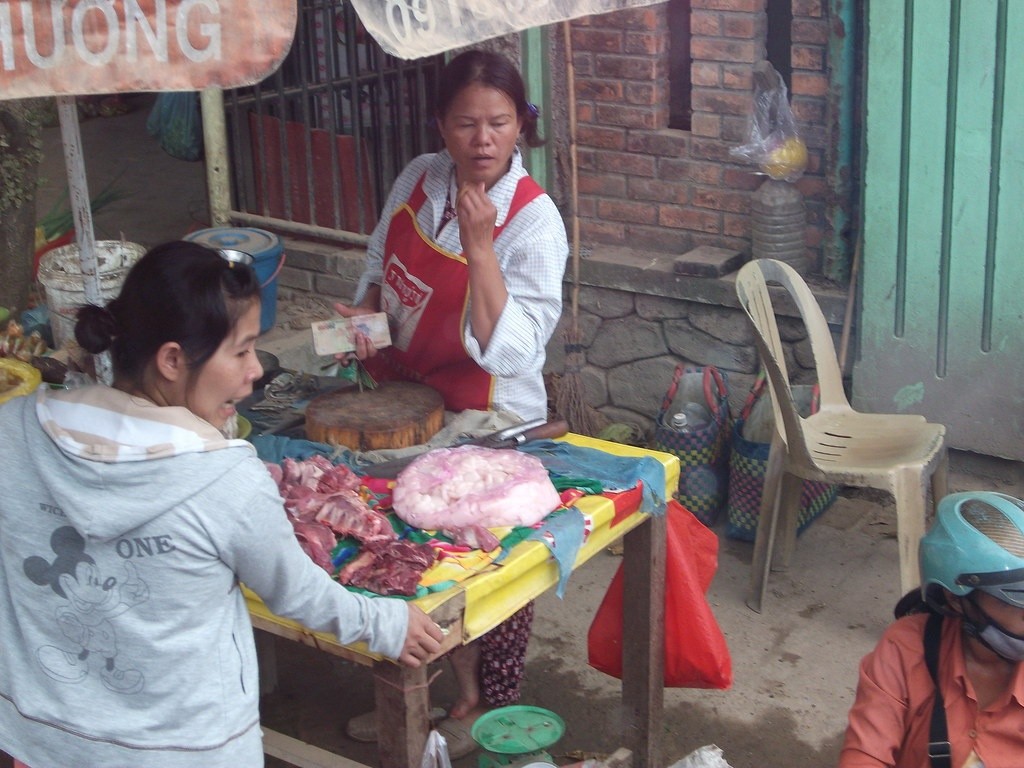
[472,705,565,767]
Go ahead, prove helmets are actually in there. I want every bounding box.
[918,491,1024,609]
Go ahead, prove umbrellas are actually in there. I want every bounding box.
[1,1,671,398]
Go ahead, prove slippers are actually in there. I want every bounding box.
[349,700,447,742]
[436,710,488,759]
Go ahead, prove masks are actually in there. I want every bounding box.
[956,596,1024,663]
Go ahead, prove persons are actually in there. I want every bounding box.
[0,239,443,768]
[334,49,570,721]
[839,491,1024,768]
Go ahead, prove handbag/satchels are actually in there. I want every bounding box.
[652,365,728,525]
[729,369,839,543]
[586,499,732,690]
[729,70,807,184]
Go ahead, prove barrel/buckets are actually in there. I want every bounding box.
[183,225,283,336]
[37,237,147,365]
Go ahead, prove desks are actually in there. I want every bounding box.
[237,370,680,768]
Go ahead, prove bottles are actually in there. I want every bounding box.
[750,176,808,286]
[671,403,710,435]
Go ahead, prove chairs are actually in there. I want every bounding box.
[735,257,949,614]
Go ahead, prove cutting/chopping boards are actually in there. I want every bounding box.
[304,378,446,451]
